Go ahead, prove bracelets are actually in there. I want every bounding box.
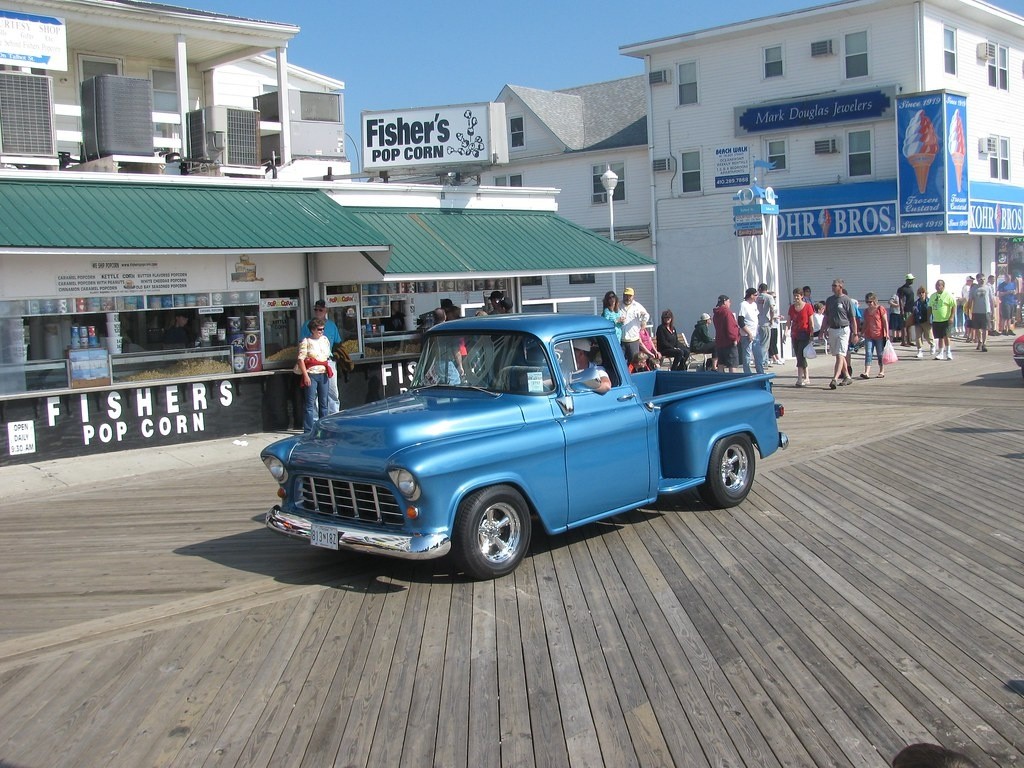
[820,329,824,333]
[854,334,858,336]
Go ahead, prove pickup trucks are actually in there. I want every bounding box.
[259,310,790,582]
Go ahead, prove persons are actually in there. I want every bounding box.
[544,338,612,393]
[299,300,342,425]
[738,287,767,374]
[379,301,405,331]
[104,316,144,353]
[962,272,1018,350]
[164,310,191,342]
[785,287,814,386]
[802,285,862,352]
[713,295,740,373]
[690,312,719,372]
[433,299,465,385]
[601,287,663,374]
[927,279,956,359]
[859,292,891,378]
[819,280,859,388]
[656,308,690,371]
[475,290,525,386]
[757,283,784,370]
[298,317,334,435]
[888,274,935,357]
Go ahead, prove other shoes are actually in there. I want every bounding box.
[839,377,852,386]
[803,380,811,384]
[860,374,870,379]
[763,358,786,368]
[830,380,837,389]
[796,382,805,386]
[877,374,884,378]
[894,328,1016,360]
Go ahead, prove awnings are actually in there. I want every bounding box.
[342,203,657,282]
[0,178,395,255]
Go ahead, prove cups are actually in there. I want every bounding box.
[201,322,225,341]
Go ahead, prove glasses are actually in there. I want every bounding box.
[314,309,323,311]
[608,296,615,299]
[866,301,873,303]
[315,327,324,331]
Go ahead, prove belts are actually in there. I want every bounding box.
[830,326,846,328]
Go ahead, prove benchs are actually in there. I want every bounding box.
[500,366,554,393]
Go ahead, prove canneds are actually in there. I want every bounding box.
[71,325,97,349]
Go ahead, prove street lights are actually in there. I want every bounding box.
[599,164,619,294]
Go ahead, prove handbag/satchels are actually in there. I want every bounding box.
[882,340,898,364]
[803,342,817,359]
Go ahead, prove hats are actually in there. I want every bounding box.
[558,337,590,352]
[716,295,729,307]
[488,291,504,299]
[623,288,634,295]
[314,299,325,307]
[701,313,711,320]
[966,276,975,280]
[743,288,756,299]
[905,274,915,280]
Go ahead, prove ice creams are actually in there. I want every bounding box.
[995,204,1002,232]
[948,109,965,193]
[902,109,939,193]
[817,209,831,237]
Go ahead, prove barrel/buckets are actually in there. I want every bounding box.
[105,313,122,354]
[8,319,28,362]
[227,315,263,373]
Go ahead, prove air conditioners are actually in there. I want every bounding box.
[649,69,669,86]
[814,138,838,154]
[980,138,997,154]
[811,40,833,56]
[978,43,995,59]
[653,159,674,172]
